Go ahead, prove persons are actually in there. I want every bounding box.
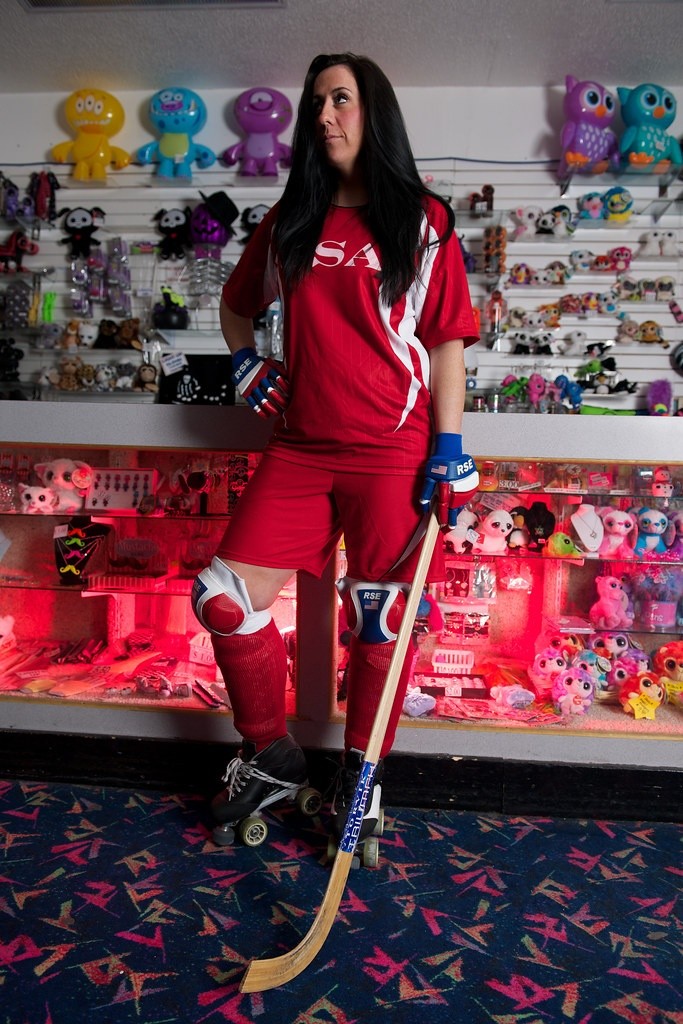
[190,54,479,866]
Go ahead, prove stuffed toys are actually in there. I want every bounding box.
[507,186,679,357]
[52,207,106,261]
[35,318,158,392]
[443,508,529,555]
[646,379,672,416]
[153,207,191,260]
[18,459,93,513]
[500,357,638,408]
[590,576,633,629]
[240,205,271,244]
[533,631,683,715]
[596,504,683,559]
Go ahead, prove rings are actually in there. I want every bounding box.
[91,474,149,507]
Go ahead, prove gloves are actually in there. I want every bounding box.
[419,433,480,531]
[231,347,290,420]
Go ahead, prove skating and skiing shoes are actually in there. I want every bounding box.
[212,732,323,847]
[327,747,385,868]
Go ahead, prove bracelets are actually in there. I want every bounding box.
[232,347,255,367]
[434,433,463,456]
[444,623,489,639]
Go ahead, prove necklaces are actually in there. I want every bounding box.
[576,512,597,539]
[57,521,105,575]
[534,513,548,535]
